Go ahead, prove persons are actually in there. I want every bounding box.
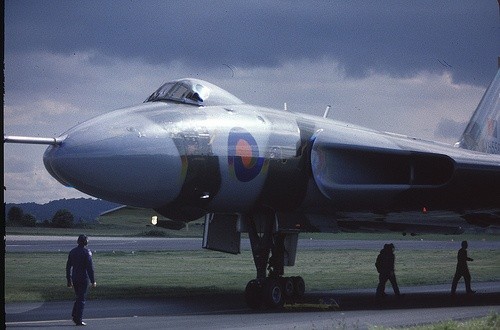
[450,241,477,297]
[375,243,401,300]
[65,235,98,325]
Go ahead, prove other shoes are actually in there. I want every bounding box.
[72,319,87,326]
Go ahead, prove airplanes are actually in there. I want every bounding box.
[5,59,500,310]
